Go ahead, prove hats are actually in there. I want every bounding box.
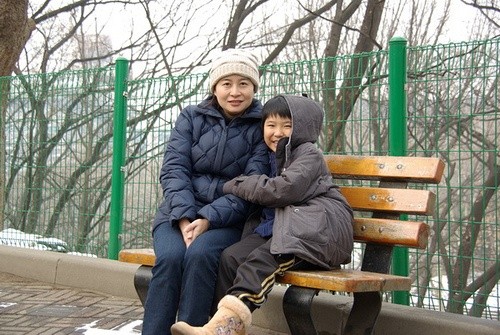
[210,49,261,92]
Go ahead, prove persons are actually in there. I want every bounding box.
[171,91,356,335]
[141,48,266,335]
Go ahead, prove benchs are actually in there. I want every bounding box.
[117,155,445,335]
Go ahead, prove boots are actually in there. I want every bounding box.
[171,294,253,334]
